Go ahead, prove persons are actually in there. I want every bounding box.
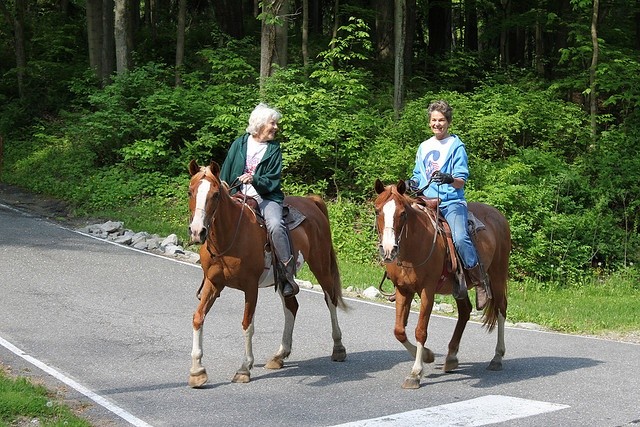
[218,103,300,297]
[409,100,492,310]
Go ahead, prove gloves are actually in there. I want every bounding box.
[432,172,454,186]
[404,179,419,190]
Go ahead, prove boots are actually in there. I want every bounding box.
[464,262,488,310]
[279,262,294,297]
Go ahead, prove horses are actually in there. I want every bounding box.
[373,178,513,392]
[187,158,357,391]
